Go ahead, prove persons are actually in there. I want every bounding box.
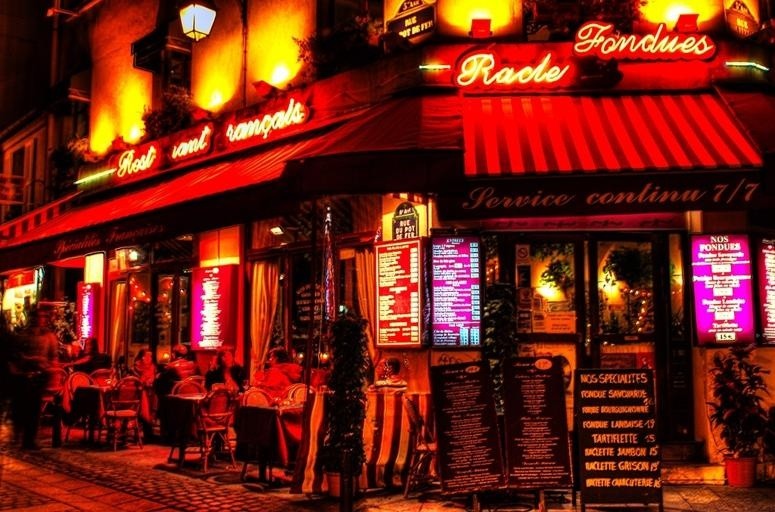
[3,310,406,458]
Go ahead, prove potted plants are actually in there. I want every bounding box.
[706,346,768,489]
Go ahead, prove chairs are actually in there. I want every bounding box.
[41,367,317,486]
[400,393,440,498]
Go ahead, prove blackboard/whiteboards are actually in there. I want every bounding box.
[429,355,568,494]
[574,366,662,501]
